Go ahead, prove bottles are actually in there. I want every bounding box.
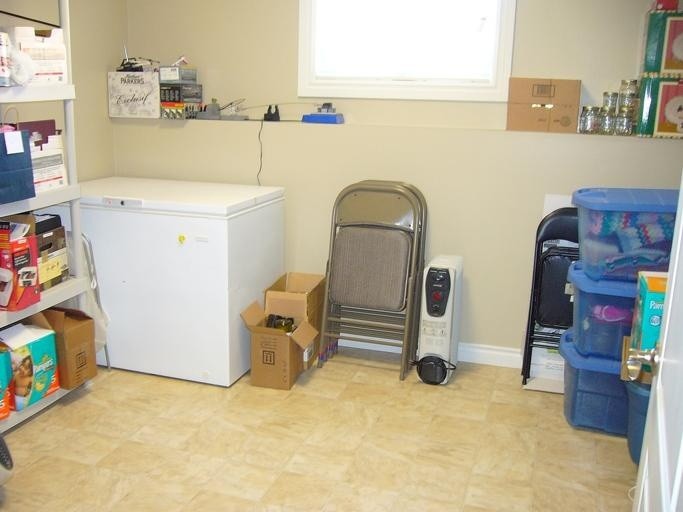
[580,80,639,136]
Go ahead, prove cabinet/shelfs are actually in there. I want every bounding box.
[0,1,97,438]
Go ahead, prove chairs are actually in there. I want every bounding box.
[522,207,580,385]
[316,180,427,380]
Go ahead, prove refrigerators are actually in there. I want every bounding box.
[38,175,285,388]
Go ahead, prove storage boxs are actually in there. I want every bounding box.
[506,77,580,133]
[0,328,60,418]
[0,130,36,205]
[640,10,683,75]
[629,75,683,140]
[107,70,161,119]
[241,272,327,390]
[36,215,70,291]
[559,189,683,435]
[30,134,68,193]
[0,214,41,311]
[33,307,99,389]
[2,27,67,86]
